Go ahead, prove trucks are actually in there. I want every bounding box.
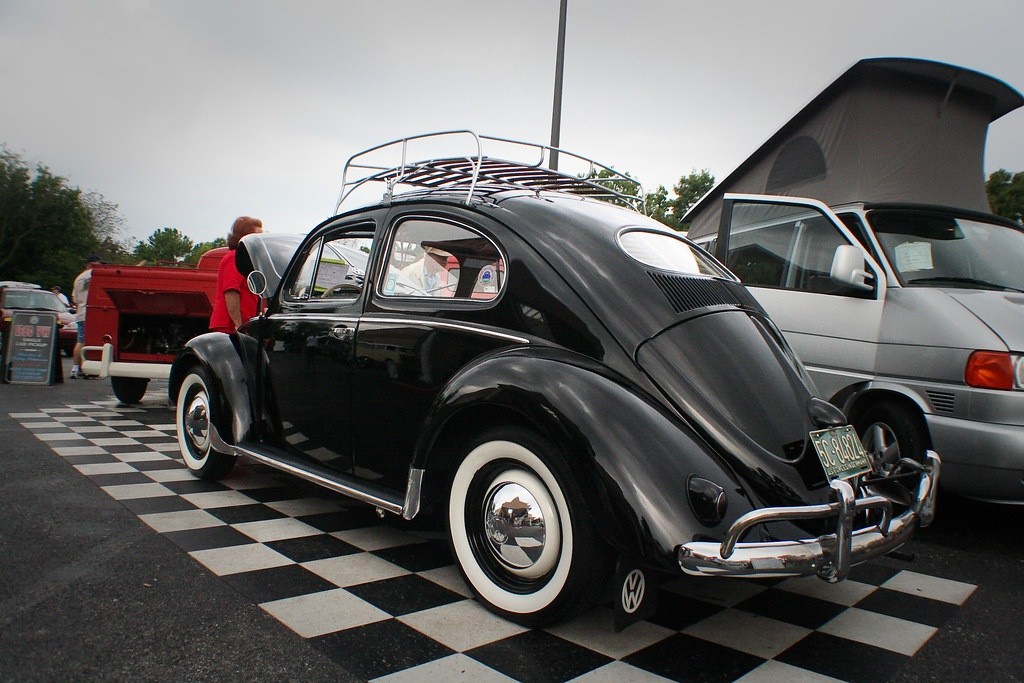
[83,246,268,406]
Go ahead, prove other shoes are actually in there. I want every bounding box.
[70,371,80,378]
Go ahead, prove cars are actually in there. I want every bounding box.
[651,56,1024,511]
[170,131,940,628]
[0,288,78,357]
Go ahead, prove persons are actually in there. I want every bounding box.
[69,255,148,378]
[209,217,267,335]
[51,286,70,308]
[395,246,453,296]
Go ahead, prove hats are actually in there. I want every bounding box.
[50,286,61,291]
[87,255,108,264]
[424,246,453,257]
